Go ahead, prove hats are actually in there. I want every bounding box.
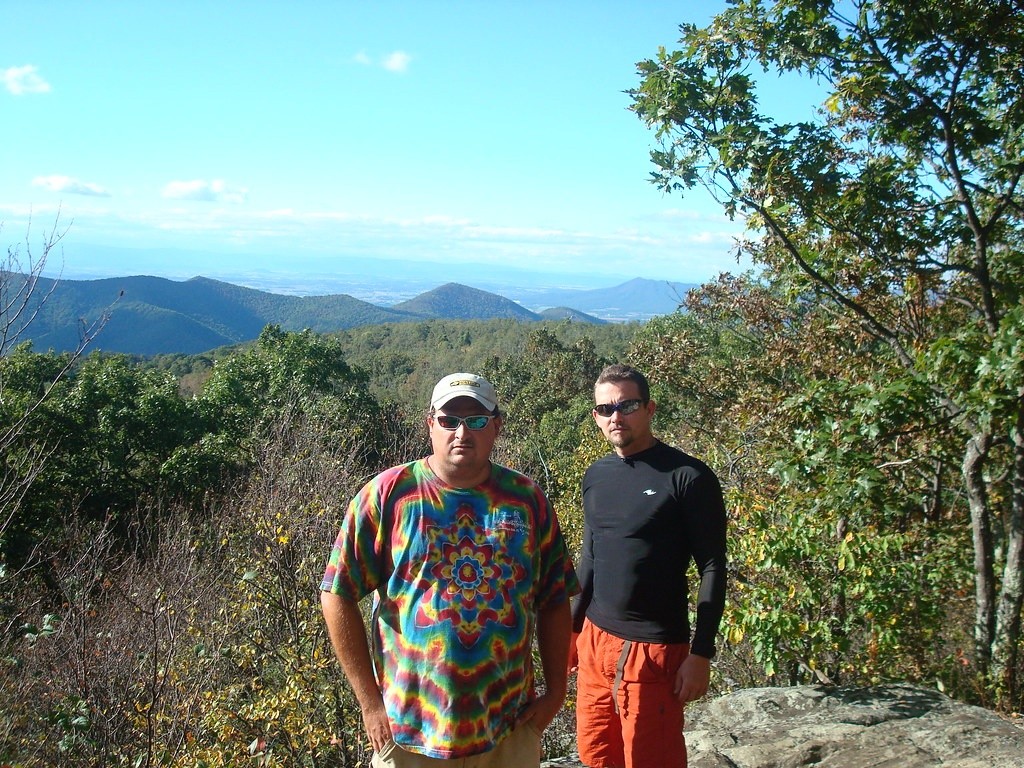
[430,373,497,414]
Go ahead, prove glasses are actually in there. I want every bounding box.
[432,415,495,430]
[595,399,645,417]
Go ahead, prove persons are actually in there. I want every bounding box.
[318,372,583,768]
[567,363,728,768]
